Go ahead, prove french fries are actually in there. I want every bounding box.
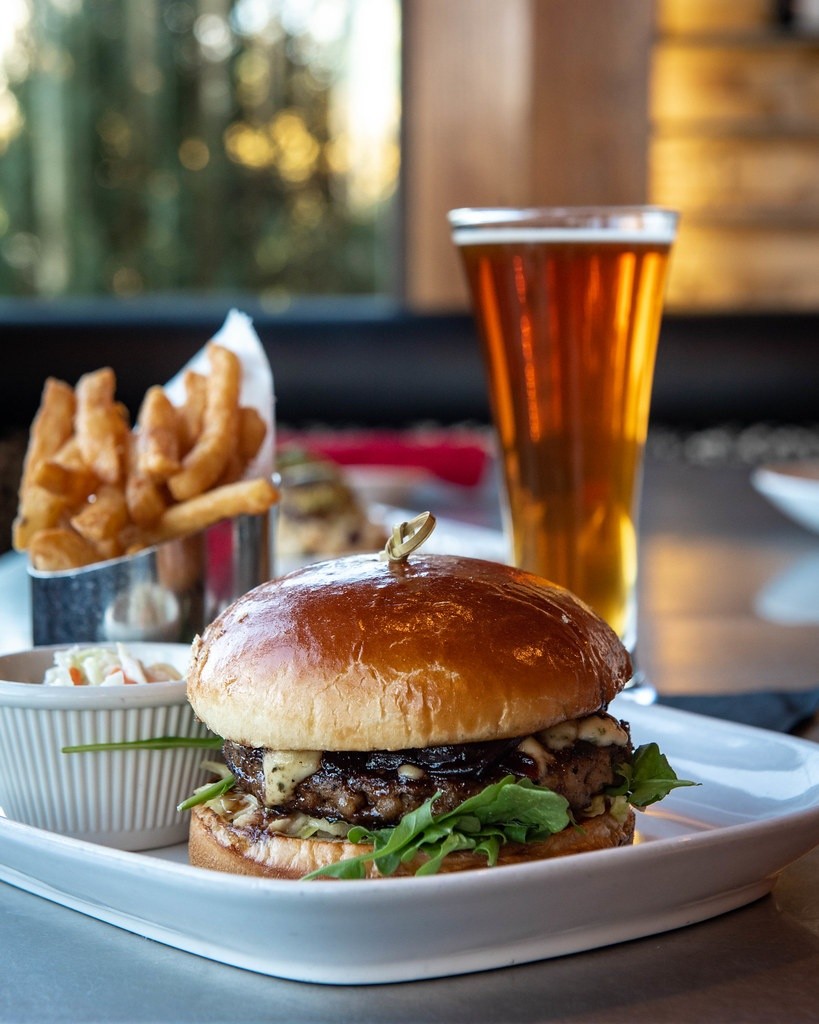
[11,347,277,569]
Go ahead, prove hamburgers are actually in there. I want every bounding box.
[189,512,701,881]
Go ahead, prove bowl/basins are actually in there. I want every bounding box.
[0,640,223,851]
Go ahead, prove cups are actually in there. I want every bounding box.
[29,473,281,647]
[446,205,676,704]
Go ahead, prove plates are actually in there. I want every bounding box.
[0,706,819,988]
